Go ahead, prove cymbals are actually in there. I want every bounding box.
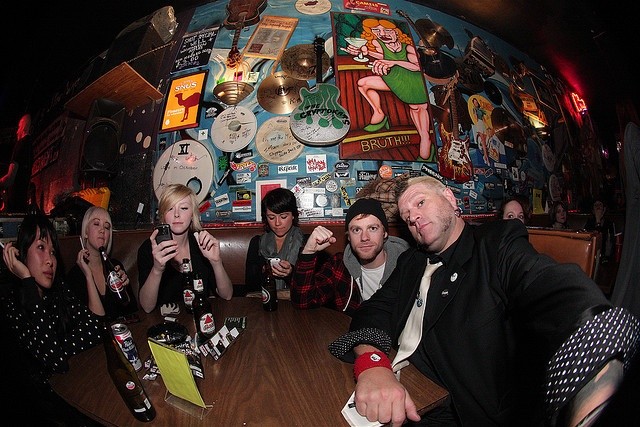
[256,71,310,115]
[415,18,455,51]
[280,43,331,81]
[483,81,503,106]
[491,107,525,150]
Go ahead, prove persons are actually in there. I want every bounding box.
[328,176,640,427]
[136,184,234,314]
[496,198,533,226]
[245,188,304,291]
[62,206,139,324]
[290,197,411,317]
[47,192,94,223]
[583,199,617,280]
[550,200,576,229]
[339,18,432,160]
[472,98,491,167]
[1,113,34,213]
[0,213,102,399]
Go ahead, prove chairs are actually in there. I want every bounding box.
[522,229,602,282]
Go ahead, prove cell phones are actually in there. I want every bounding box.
[154,224,176,256]
[268,257,281,267]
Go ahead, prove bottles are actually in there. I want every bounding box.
[180,258,196,311]
[97,245,131,308]
[259,260,276,314]
[98,330,155,420]
[191,278,216,344]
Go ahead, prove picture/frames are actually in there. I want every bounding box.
[243,17,298,60]
[329,10,439,165]
[256,179,287,222]
[528,71,558,113]
[157,69,209,133]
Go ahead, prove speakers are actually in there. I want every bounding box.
[76,97,126,176]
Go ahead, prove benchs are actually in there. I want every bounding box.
[55,225,417,315]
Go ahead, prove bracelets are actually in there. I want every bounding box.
[352,351,393,381]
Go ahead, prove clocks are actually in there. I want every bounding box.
[152,139,214,209]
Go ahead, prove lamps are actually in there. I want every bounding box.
[212,80,253,105]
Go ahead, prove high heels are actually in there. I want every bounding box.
[416,143,437,162]
[363,114,389,133]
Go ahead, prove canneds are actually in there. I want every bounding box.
[110,323,143,373]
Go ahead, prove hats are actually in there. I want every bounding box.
[345,198,388,232]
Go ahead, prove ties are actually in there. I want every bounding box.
[341,257,444,427]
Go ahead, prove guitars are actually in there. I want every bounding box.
[289,37,351,148]
[438,91,474,184]
[223,0,267,69]
[396,9,457,79]
